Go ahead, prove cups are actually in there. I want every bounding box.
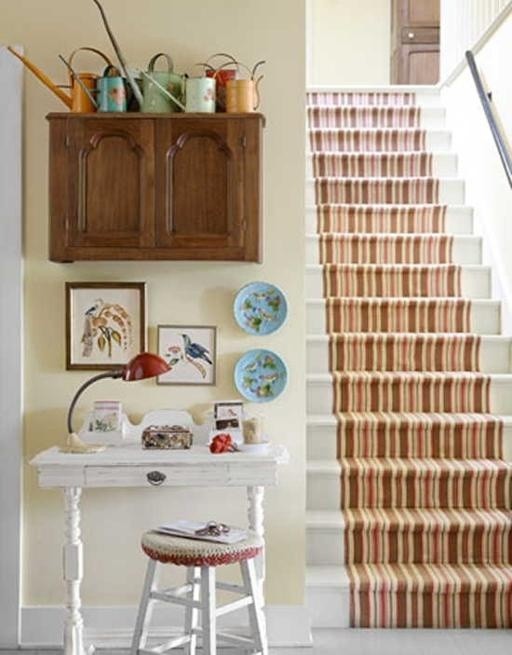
[243,419,266,444]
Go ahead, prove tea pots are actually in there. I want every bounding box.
[4,0,267,117]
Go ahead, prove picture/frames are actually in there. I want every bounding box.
[64,280,149,371]
[154,324,218,387]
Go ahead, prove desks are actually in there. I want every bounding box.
[26,439,290,655]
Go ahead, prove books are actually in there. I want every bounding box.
[153,519,249,545]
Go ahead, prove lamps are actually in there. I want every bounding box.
[59,350,172,456]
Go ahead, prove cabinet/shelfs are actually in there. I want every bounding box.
[42,111,268,266]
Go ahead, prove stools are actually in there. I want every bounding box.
[128,522,270,655]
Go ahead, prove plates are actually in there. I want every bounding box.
[232,348,288,404]
[233,280,288,336]
[237,441,272,453]
